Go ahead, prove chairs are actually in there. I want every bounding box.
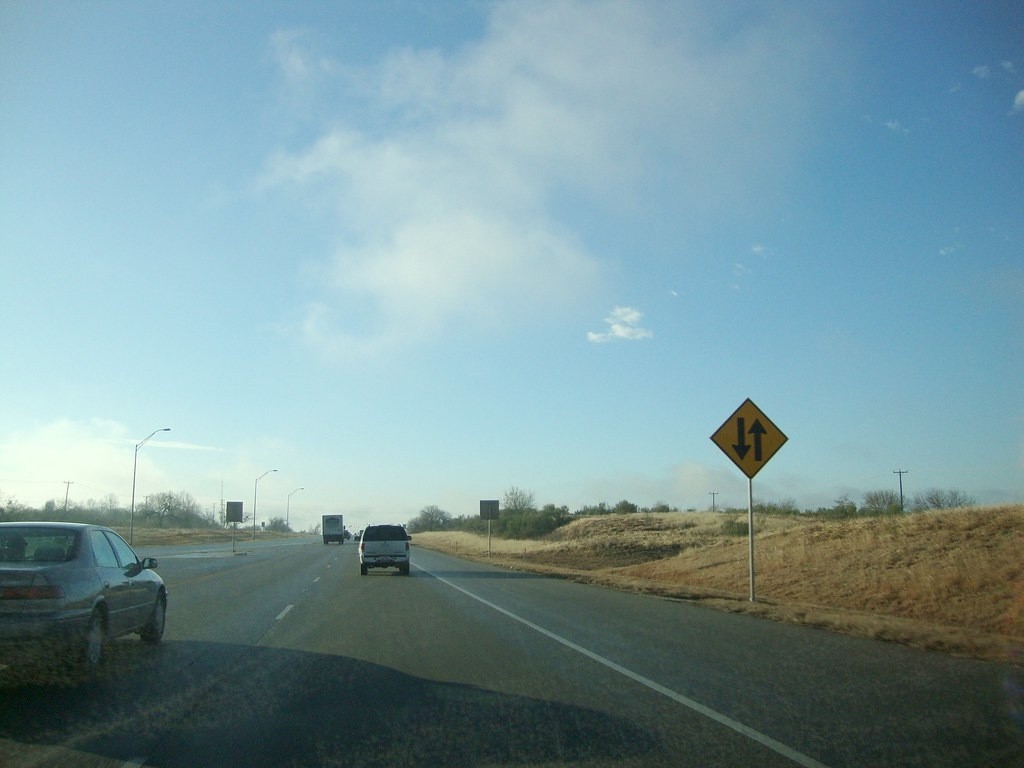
[33,547,68,562]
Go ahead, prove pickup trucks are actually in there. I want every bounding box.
[358,525,412,575]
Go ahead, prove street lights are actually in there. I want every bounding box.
[129,428,171,546]
[286,487,304,538]
[253,469,277,539]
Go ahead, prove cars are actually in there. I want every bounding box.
[344,531,350,540]
[0,521,168,670]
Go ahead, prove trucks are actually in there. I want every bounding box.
[322,515,346,544]
